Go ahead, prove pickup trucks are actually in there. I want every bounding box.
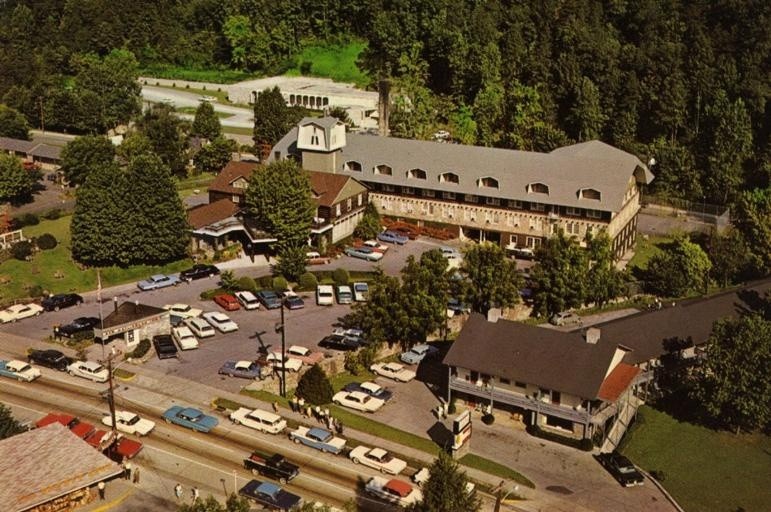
[244,451,299,484]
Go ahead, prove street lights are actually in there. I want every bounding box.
[273,291,298,399]
[499,485,520,511]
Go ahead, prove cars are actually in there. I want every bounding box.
[234,290,260,311]
[354,240,389,254]
[274,289,305,310]
[179,263,221,283]
[229,407,287,435]
[317,334,360,352]
[161,303,203,319]
[0,288,157,462]
[255,290,282,310]
[213,293,240,311]
[203,311,239,334]
[438,246,472,319]
[599,450,645,489]
[256,352,304,374]
[152,334,179,360]
[218,360,263,383]
[400,344,439,365]
[171,325,200,351]
[348,446,405,476]
[289,426,345,456]
[136,274,182,291]
[182,317,216,339]
[331,390,386,413]
[239,481,300,511]
[353,282,369,302]
[364,477,424,508]
[414,467,476,501]
[549,312,581,328]
[369,361,417,383]
[332,328,363,343]
[343,381,394,405]
[303,252,330,265]
[316,284,334,306]
[197,95,218,102]
[344,246,383,262]
[274,344,325,366]
[435,128,461,145]
[336,285,353,304]
[377,230,409,245]
[160,405,219,433]
